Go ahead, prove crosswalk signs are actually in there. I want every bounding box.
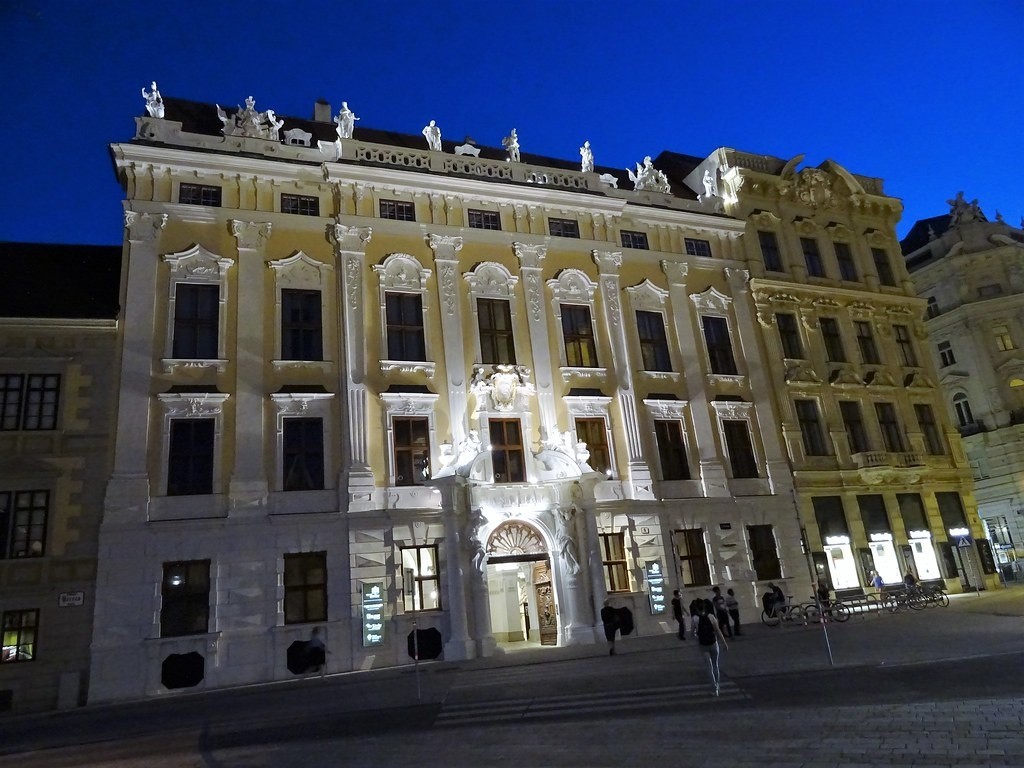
[958,536,971,548]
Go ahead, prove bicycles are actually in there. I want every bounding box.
[881,580,949,613]
[761,593,808,627]
[804,589,850,623]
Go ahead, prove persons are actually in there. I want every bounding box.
[601,598,617,656]
[422,120,441,152]
[334,102,361,138]
[870,571,885,605]
[299,626,332,680]
[691,598,728,696]
[768,583,786,621]
[818,581,835,622]
[671,589,689,641]
[726,589,741,637]
[142,81,165,118]
[502,128,520,163]
[712,586,735,641]
[626,156,672,194]
[580,141,593,173]
[1010,556,1020,584]
[905,570,916,599]
[216,96,284,141]
[702,170,713,198]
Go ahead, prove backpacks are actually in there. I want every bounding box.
[695,612,717,646]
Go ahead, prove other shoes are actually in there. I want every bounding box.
[735,631,742,636]
[678,635,687,640]
[609,648,616,655]
[728,634,734,637]
[710,682,720,697]
